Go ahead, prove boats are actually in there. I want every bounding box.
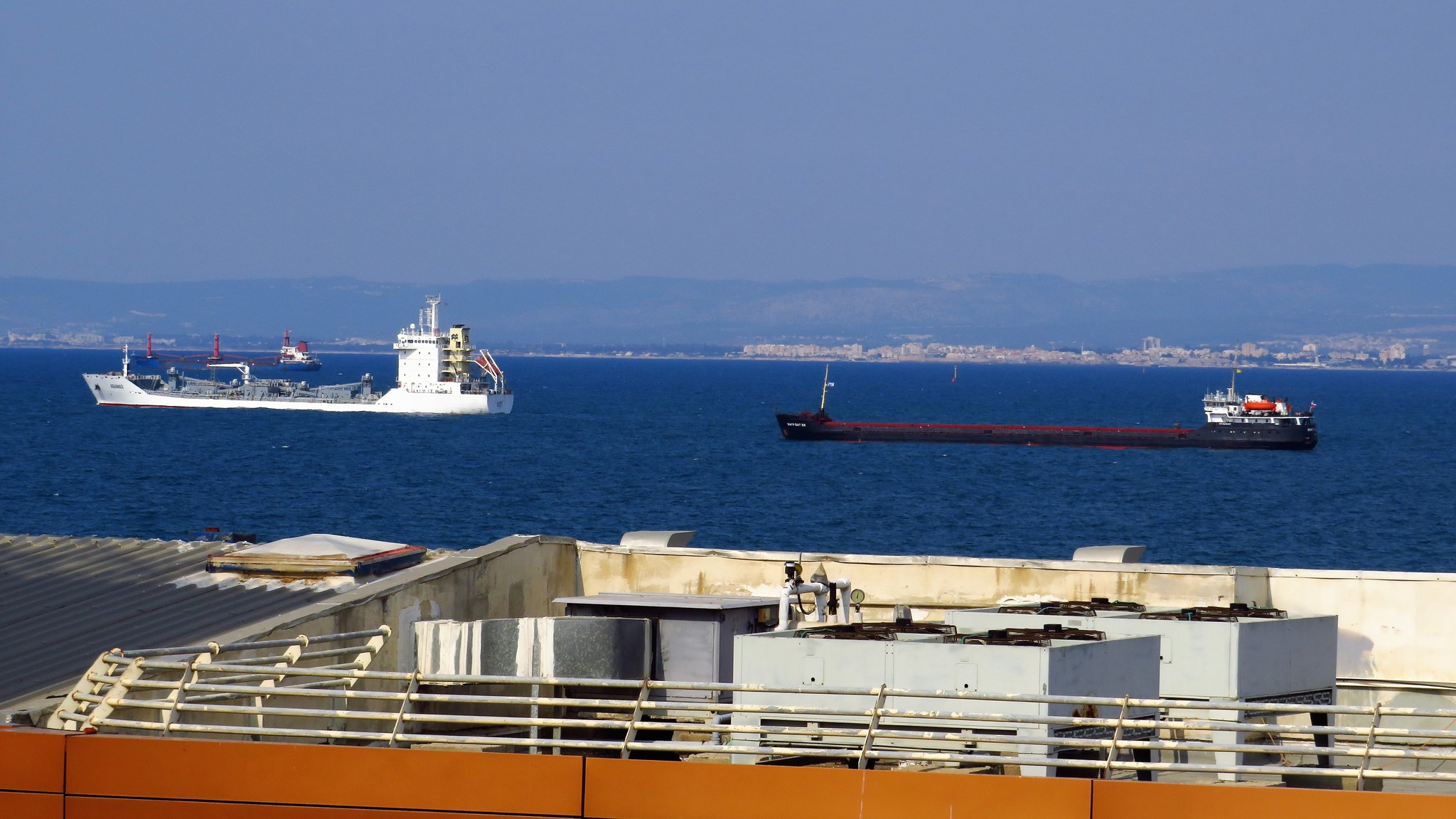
[128,327,322,371]
[777,359,1320,452]
[82,291,519,416]
